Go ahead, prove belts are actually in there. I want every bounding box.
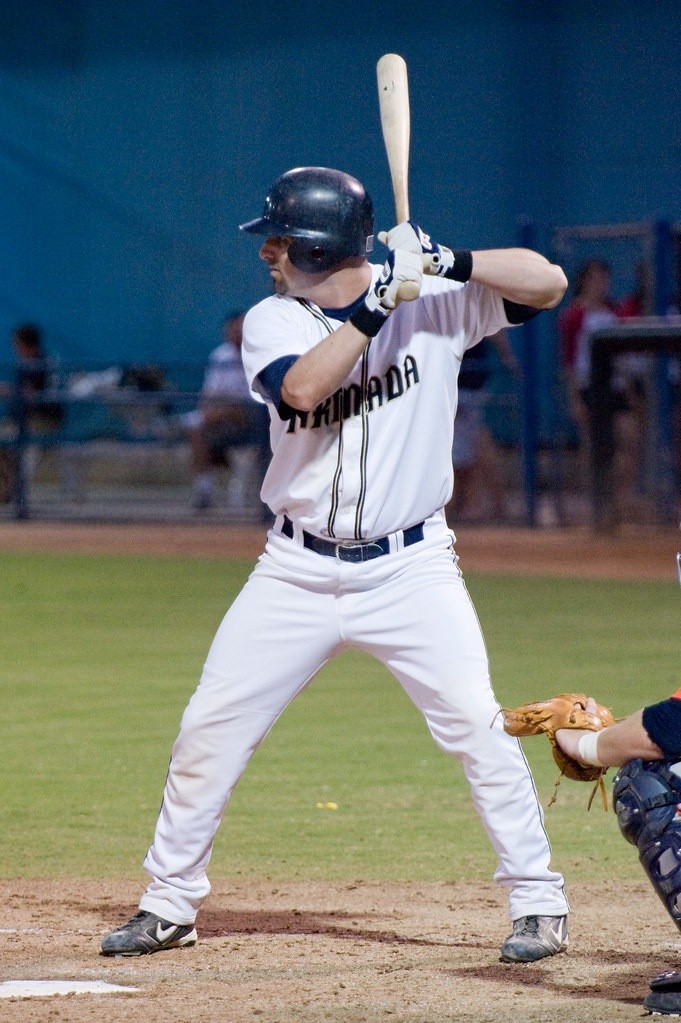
[280,514,426,563]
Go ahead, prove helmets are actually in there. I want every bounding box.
[239,166,376,274]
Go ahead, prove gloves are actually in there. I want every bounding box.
[377,220,455,278]
[365,250,422,316]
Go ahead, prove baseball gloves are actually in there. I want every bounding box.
[502,693,616,781]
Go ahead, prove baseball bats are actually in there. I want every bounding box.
[374,51,422,300]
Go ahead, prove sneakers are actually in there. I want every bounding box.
[100,909,198,957]
[499,914,569,962]
[643,971,681,1014]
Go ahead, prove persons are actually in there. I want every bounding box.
[557,257,655,520]
[0,324,81,520]
[156,314,277,523]
[60,362,173,416]
[100,165,570,963]
[555,679,681,1013]
[535,438,561,525]
[445,327,518,521]
